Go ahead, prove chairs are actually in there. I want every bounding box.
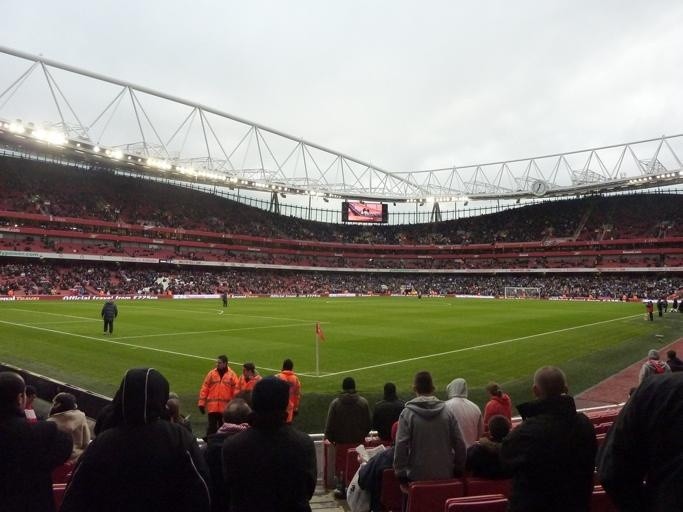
[323,406,621,512]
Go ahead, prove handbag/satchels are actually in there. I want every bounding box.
[346,461,374,512]
